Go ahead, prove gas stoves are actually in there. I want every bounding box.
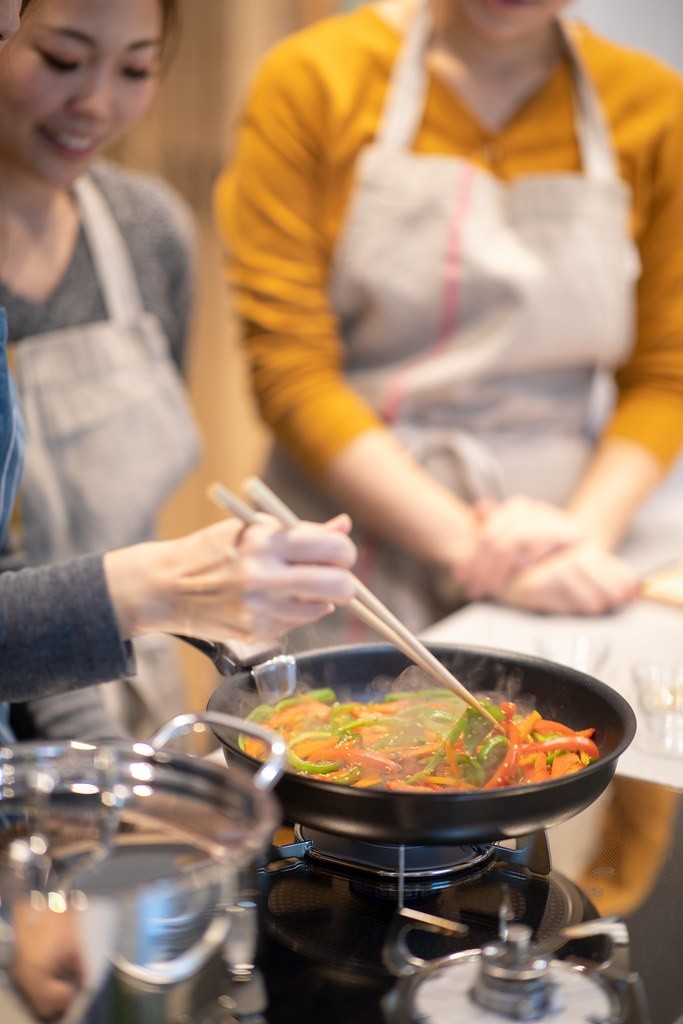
[199,744,683,1024]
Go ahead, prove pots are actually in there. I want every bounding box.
[0,712,282,1024]
[169,634,635,843]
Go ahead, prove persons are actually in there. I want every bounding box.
[209,0,683,657]
[0,0,357,751]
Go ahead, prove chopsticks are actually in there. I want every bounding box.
[205,477,505,737]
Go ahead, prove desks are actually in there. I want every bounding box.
[417,458,683,788]
